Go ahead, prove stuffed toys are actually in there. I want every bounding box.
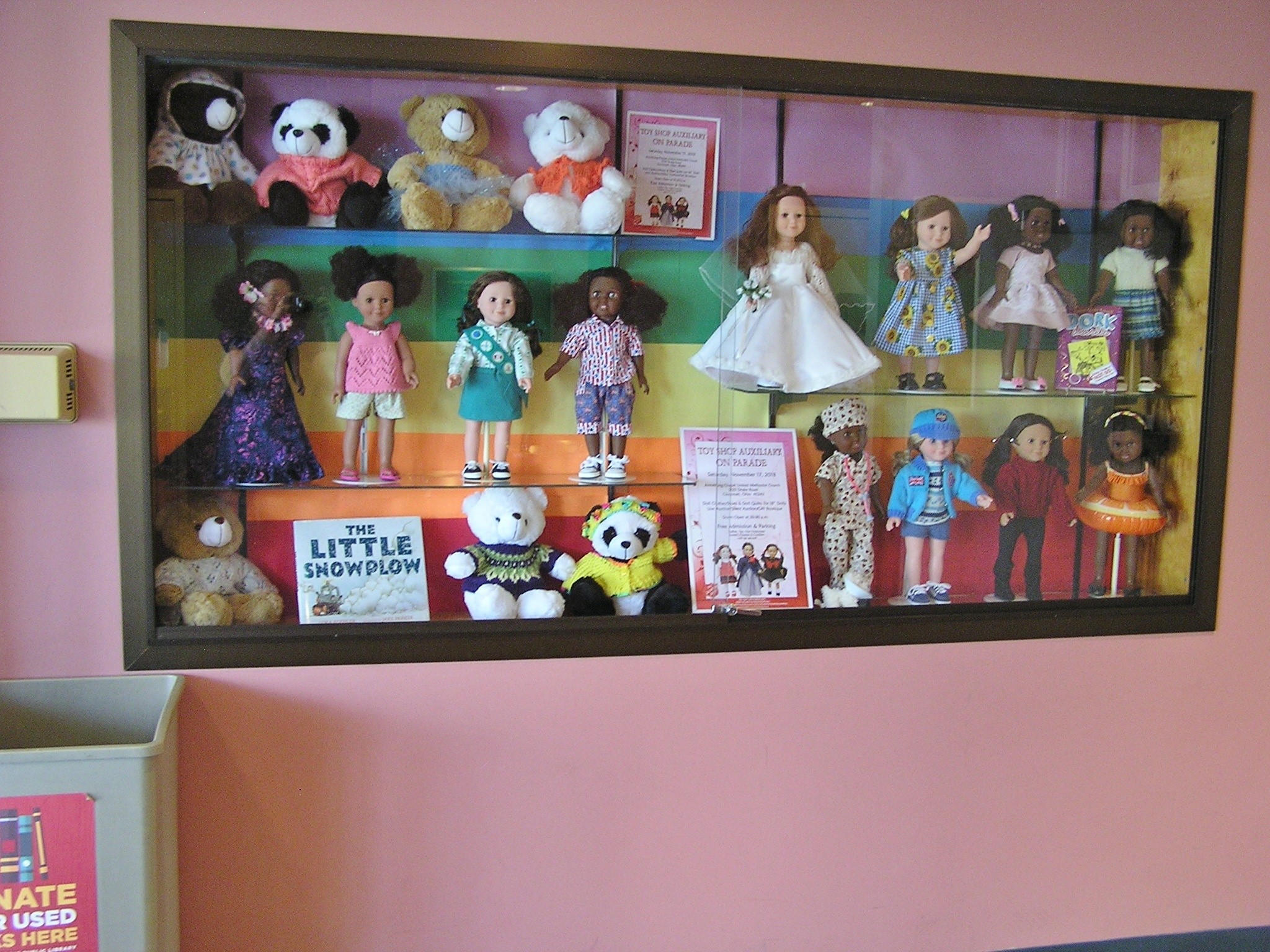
[560,495,690,616]
[146,65,262,227]
[252,97,390,230]
[444,485,576,620]
[508,100,635,234]
[152,490,284,628]
[387,93,514,232]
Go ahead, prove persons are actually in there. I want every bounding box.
[885,408,993,605]
[968,194,1080,391]
[329,245,423,481]
[871,194,993,389]
[1089,200,1181,393]
[152,260,325,485]
[448,270,542,479]
[807,397,887,594]
[979,413,1078,601]
[713,542,787,597]
[1074,406,1176,596]
[545,265,669,480]
[690,183,884,394]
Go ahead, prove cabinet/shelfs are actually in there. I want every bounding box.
[142,67,1199,600]
